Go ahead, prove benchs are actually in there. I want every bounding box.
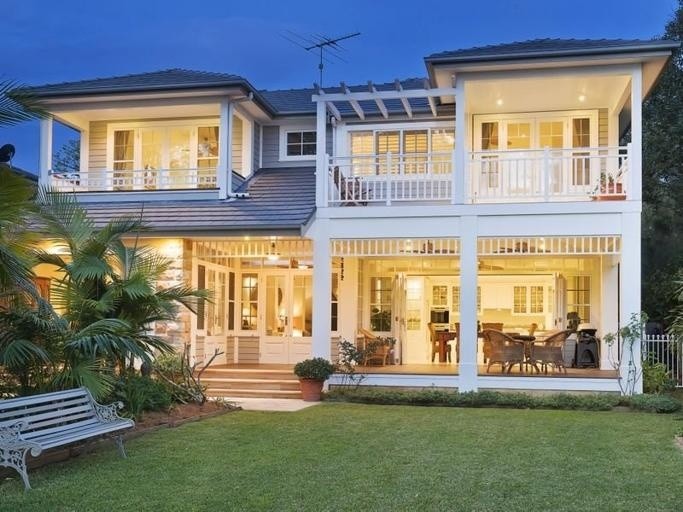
[1,385,136,488]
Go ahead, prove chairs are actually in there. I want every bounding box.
[428,321,574,375]
[334,166,371,205]
[359,327,391,367]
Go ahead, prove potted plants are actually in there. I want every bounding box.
[294,357,335,401]
[595,172,622,193]
